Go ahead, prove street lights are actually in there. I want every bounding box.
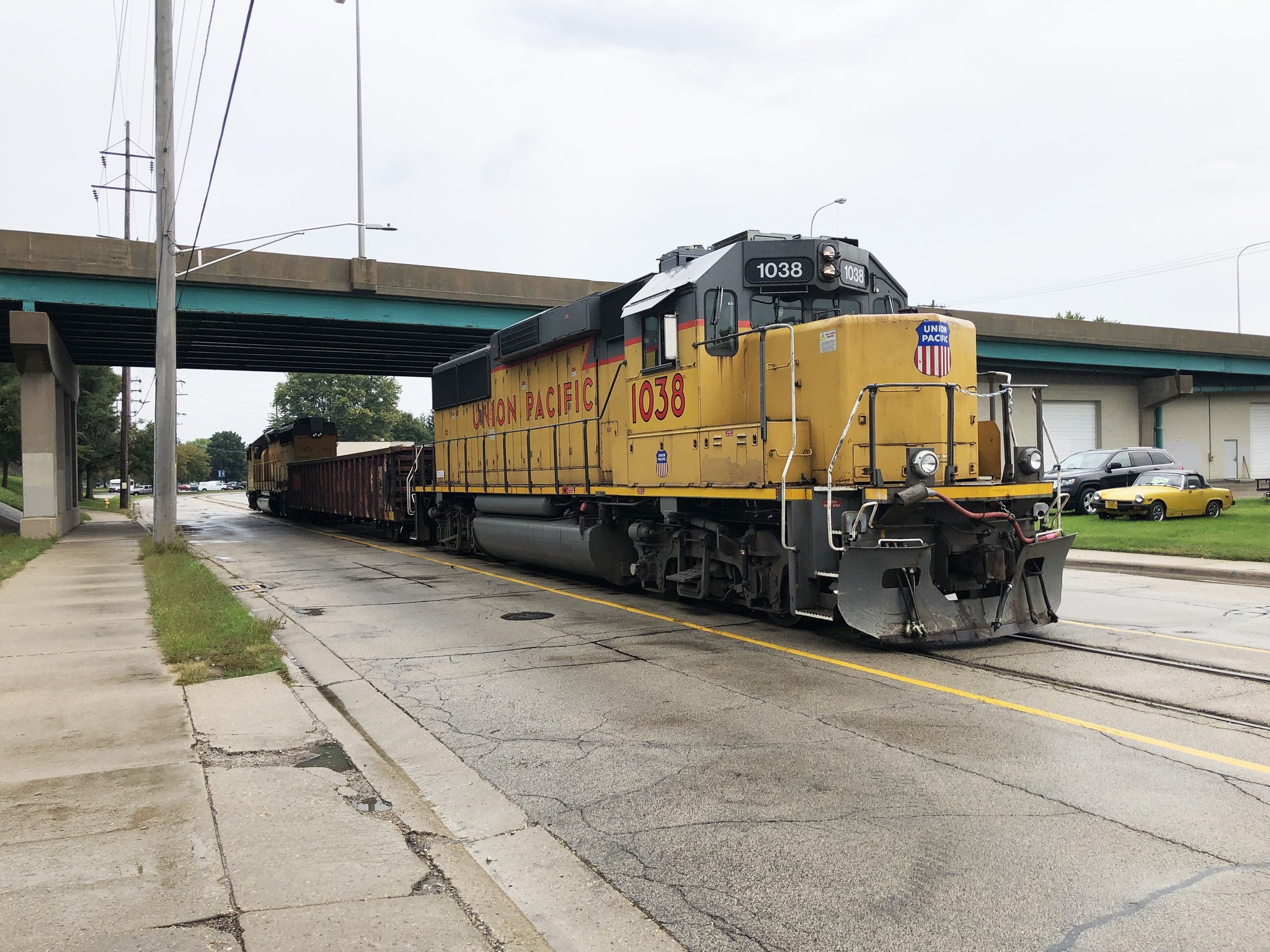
[152,223,398,547]
[334,0,366,257]
[810,197,847,237]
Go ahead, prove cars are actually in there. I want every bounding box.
[222,482,247,491]
[178,482,200,492]
[1090,471,1236,521]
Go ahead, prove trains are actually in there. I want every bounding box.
[245,230,1077,647]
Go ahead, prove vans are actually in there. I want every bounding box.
[198,481,227,492]
[108,479,134,493]
[130,485,153,496]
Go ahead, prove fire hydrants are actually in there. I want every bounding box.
[103,498,110,509]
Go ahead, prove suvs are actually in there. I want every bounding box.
[1039,447,1184,515]
